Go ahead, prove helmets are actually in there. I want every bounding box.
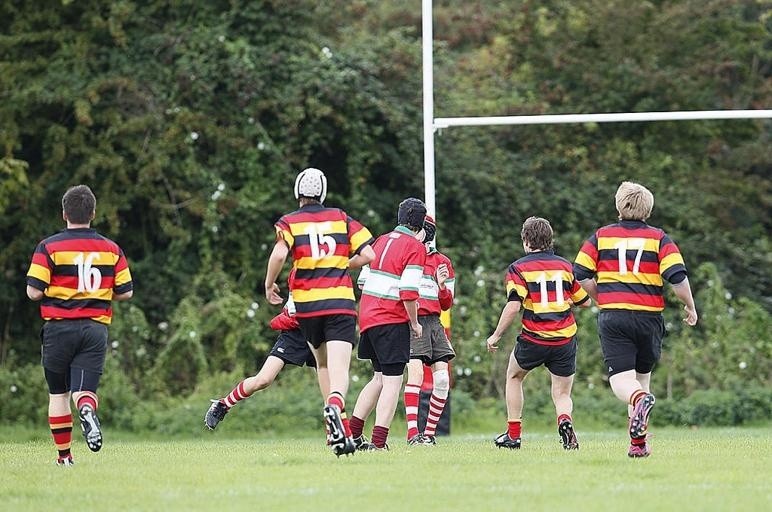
[397,197,427,230]
[292,167,328,204]
[420,214,438,245]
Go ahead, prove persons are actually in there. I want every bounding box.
[572,181,698,458]
[348,198,427,452]
[205,267,333,446]
[404,214,455,447]
[265,168,377,458]
[26,185,135,466]
[486,216,591,448]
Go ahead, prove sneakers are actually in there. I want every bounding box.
[78,403,103,453]
[493,432,521,451]
[627,443,652,460]
[54,456,75,468]
[203,396,229,431]
[628,393,656,440]
[321,403,358,456]
[558,418,579,451]
[367,441,391,453]
[354,433,370,454]
[407,432,437,447]
